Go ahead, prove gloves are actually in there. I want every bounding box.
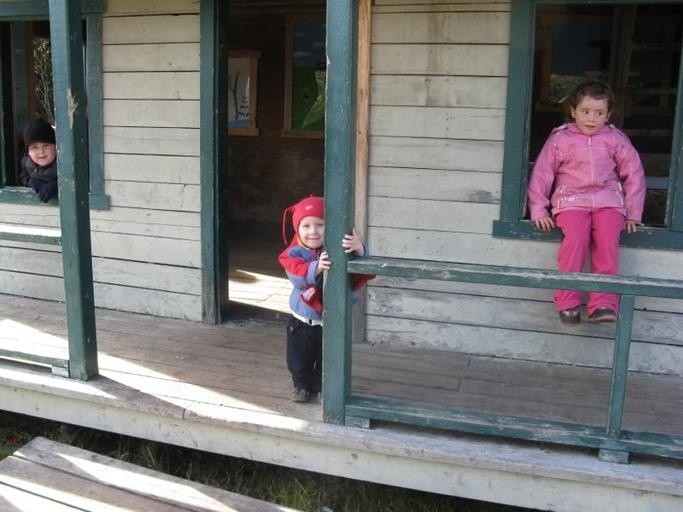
[28,179,58,203]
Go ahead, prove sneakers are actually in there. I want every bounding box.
[559,306,581,328]
[290,387,310,402]
[590,306,617,322]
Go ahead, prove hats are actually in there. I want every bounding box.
[281,196,326,245]
[25,117,55,150]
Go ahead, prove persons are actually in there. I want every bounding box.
[277,197,374,403]
[17,120,57,204]
[528,82,647,324]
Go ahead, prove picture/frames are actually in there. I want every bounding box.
[533,9,683,118]
[227,48,262,137]
[279,12,325,140]
